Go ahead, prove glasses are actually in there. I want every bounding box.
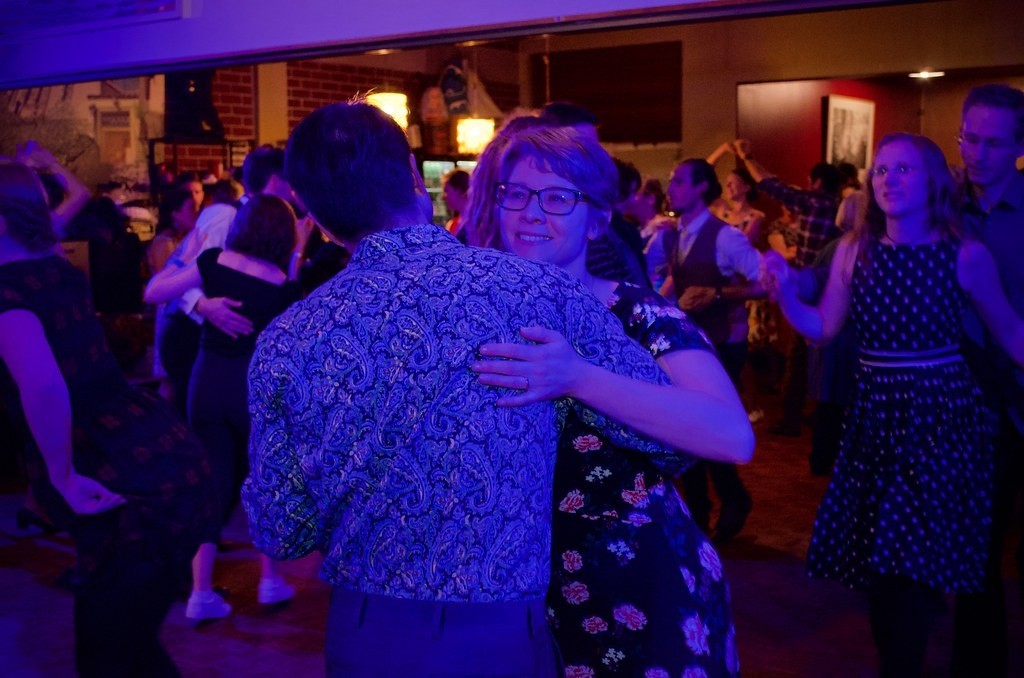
[493,182,603,215]
[874,163,910,176]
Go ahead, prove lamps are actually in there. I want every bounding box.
[365,56,409,133]
[456,46,495,154]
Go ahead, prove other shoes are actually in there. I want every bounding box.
[258,584,295,603]
[770,413,801,437]
[187,596,232,619]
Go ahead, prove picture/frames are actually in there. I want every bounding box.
[826,95,875,183]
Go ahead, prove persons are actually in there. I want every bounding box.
[240,103,702,678]
[0,86,1024,678]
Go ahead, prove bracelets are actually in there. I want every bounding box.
[290,252,303,259]
[174,259,184,268]
[715,287,722,304]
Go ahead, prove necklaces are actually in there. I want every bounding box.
[884,228,919,246]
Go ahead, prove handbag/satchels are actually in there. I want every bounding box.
[61,240,90,282]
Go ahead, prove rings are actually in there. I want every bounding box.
[523,375,529,391]
